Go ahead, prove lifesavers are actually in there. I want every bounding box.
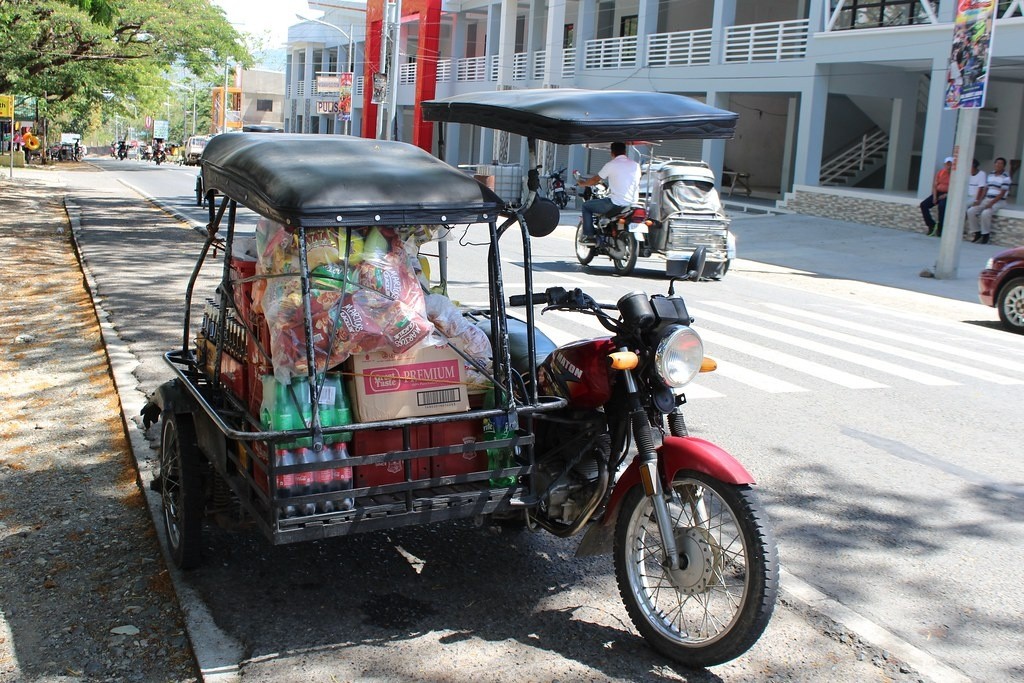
[22,132,40,150]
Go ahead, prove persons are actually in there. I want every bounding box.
[75,139,79,161]
[920,157,1011,244]
[577,143,640,246]
[110,138,178,165]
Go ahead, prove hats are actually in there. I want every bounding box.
[943,157,953,164]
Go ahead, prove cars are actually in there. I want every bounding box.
[978,244,1024,332]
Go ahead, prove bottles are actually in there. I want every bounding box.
[484,375,517,488]
[201,299,248,359]
[251,369,354,511]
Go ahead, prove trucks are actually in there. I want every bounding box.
[185,134,208,164]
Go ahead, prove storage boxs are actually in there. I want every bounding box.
[343,338,470,423]
[196,255,273,493]
[430,420,488,478]
[350,424,430,488]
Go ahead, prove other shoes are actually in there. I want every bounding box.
[971,233,982,243]
[926,222,938,236]
[980,234,989,244]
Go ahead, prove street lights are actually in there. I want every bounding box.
[296,13,355,137]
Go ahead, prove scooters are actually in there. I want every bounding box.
[548,167,570,209]
[116,146,128,160]
[155,151,164,165]
[75,146,85,163]
[146,151,153,162]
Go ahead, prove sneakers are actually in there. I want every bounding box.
[578,237,596,247]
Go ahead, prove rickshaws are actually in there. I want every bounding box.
[135,129,780,666]
[570,159,736,281]
